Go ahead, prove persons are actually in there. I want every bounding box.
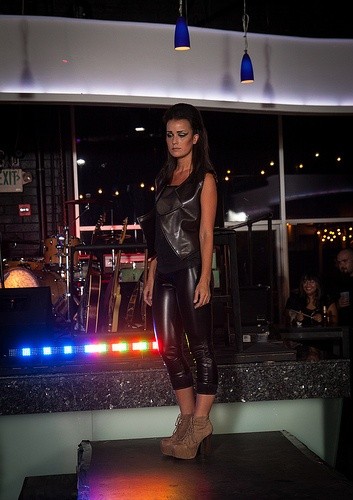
[284,271,338,328]
[144,102,217,459]
[331,248,353,327]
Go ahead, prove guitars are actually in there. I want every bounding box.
[83,211,109,334]
[125,262,154,328]
[108,216,130,333]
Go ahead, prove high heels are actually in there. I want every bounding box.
[171,415,213,460]
[159,413,201,456]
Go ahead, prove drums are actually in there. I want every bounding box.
[39,234,81,272]
[0,265,69,326]
[1,255,45,273]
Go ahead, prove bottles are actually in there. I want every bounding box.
[321,305,329,328]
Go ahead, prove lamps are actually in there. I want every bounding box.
[174,0,191,52]
[238,0,255,84]
